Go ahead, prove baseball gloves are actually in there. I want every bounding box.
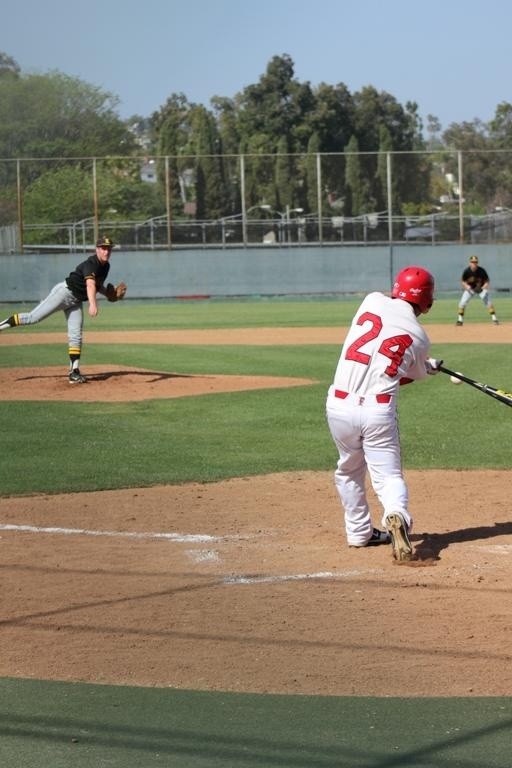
[106,282,126,301]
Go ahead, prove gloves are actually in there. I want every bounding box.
[425,357,444,375]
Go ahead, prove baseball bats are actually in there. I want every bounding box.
[440,366,511,406]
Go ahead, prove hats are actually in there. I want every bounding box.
[96,233,114,248]
[469,256,478,263]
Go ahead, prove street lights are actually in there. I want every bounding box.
[216,202,273,251]
[264,205,302,245]
[493,204,511,213]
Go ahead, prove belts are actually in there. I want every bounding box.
[335,389,391,403]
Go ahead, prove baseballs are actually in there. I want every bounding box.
[450,372,464,385]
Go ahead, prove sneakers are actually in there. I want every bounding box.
[493,320,498,324]
[456,320,462,325]
[367,527,391,544]
[67,367,85,383]
[385,510,412,560]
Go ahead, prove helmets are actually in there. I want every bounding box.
[392,264,435,314]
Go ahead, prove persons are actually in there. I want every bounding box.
[326,269,443,565]
[457,256,499,325]
[1,235,127,387]
